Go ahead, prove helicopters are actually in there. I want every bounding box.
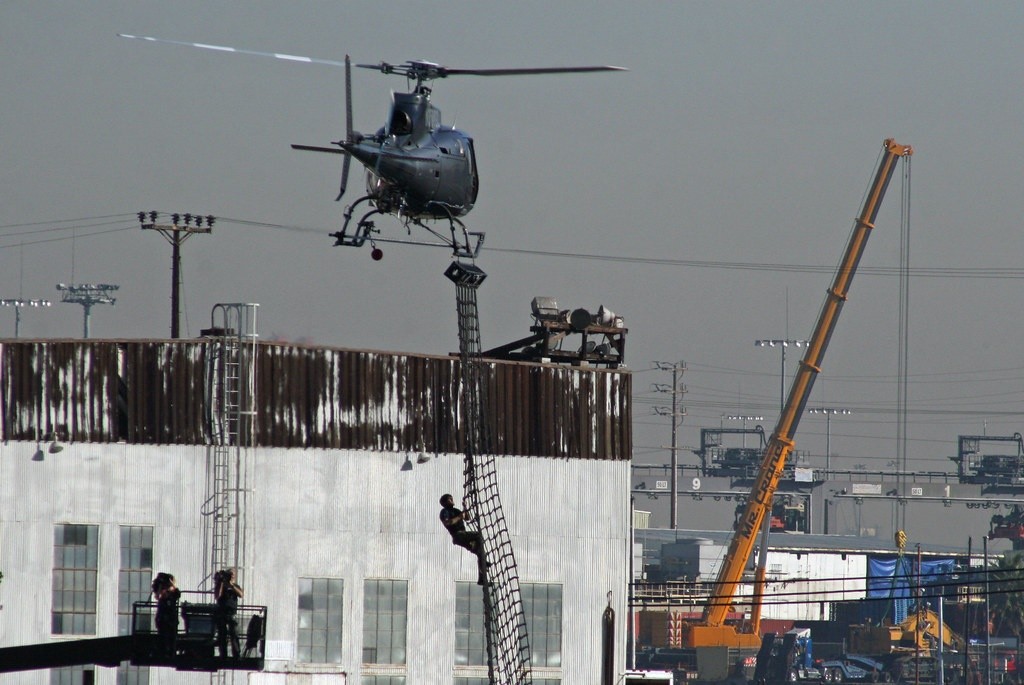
[119,32,629,275]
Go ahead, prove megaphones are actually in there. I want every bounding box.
[598,304,623,326]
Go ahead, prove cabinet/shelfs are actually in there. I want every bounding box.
[532,322,629,368]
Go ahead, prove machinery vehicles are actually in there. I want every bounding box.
[681,137,982,685]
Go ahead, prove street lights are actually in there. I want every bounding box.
[727,415,765,449]
[753,340,811,417]
[133,208,214,342]
[56,283,120,340]
[807,407,850,480]
[0,297,52,338]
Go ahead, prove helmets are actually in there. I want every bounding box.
[439,494,453,508]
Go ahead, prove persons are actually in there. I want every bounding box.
[156,568,244,660]
[439,494,494,587]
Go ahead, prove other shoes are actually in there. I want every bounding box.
[486,561,492,567]
[477,579,493,587]
